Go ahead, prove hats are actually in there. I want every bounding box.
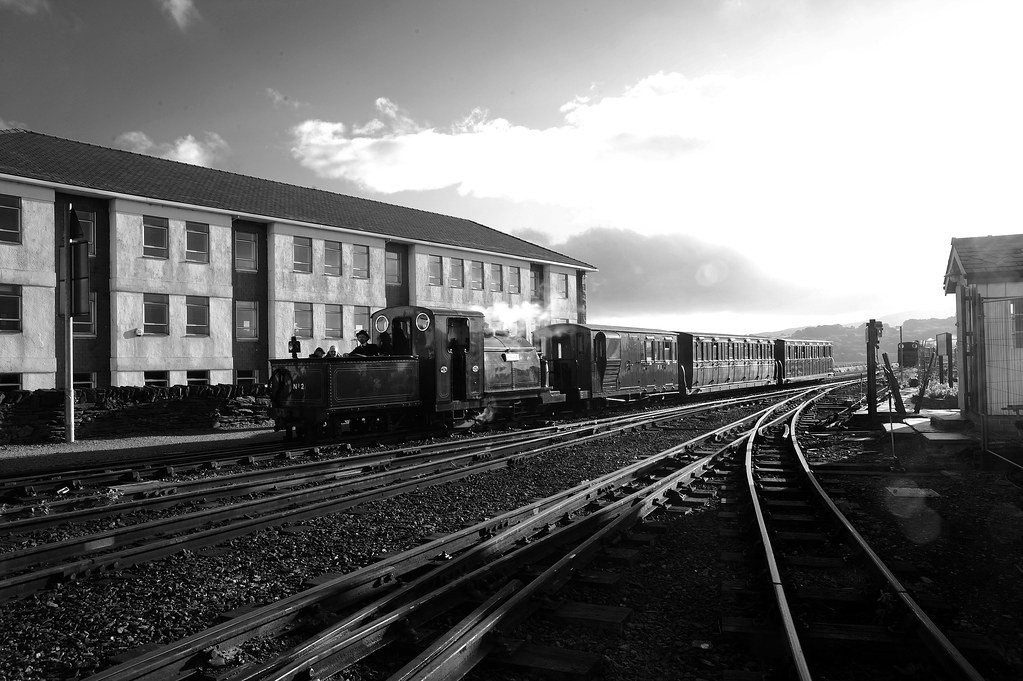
[316,347,325,354]
[356,330,369,342]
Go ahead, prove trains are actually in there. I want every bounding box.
[898,341,935,368]
[265,307,837,448]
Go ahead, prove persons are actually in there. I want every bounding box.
[323,345,341,358]
[349,330,379,356]
[309,347,325,358]
